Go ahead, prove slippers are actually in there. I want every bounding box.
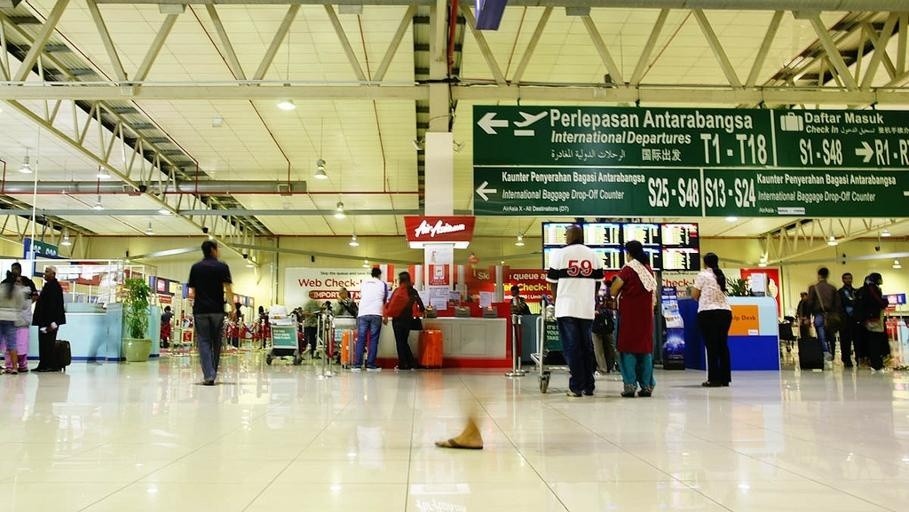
[435,440,483,449]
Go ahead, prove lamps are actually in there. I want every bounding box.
[334,165,345,220]
[313,117,326,179]
[61,226,71,246]
[94,164,104,209]
[277,31,294,110]
[892,238,902,268]
[20,146,33,174]
[363,235,370,268]
[881,216,890,237]
[515,216,525,246]
[145,213,153,235]
[349,206,360,246]
[827,217,838,245]
[758,236,767,267]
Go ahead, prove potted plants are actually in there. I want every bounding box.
[122,277,154,361]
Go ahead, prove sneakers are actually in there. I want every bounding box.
[704,381,729,387]
[621,391,635,397]
[203,380,214,385]
[638,391,651,397]
[366,368,382,372]
[567,390,581,397]
[350,366,360,371]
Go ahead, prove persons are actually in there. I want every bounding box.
[160,306,196,351]
[30,265,66,372]
[257,306,268,349]
[292,288,358,359]
[540,223,657,397]
[0,262,38,373]
[511,286,530,314]
[231,302,243,347]
[691,252,731,387]
[795,268,891,374]
[386,272,427,370]
[351,267,387,372]
[188,240,237,385]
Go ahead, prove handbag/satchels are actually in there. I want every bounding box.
[824,314,842,337]
[410,317,423,331]
[866,318,884,332]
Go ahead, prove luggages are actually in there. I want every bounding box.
[341,330,355,369]
[799,323,818,370]
[418,329,442,369]
[55,339,71,372]
